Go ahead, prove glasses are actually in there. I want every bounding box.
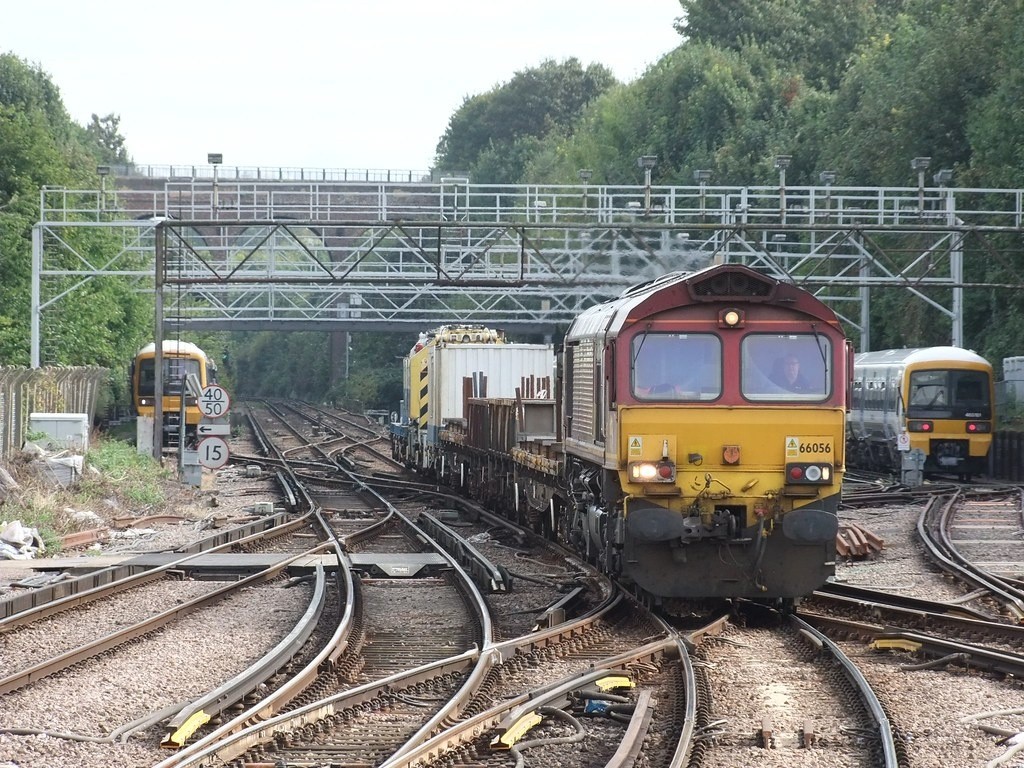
[784,362,798,365]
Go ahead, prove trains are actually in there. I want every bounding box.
[130,339,215,451]
[844,342,995,475]
[391,263,854,618]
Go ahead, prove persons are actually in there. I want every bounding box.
[766,355,819,395]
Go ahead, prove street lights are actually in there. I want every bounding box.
[96,166,111,212]
[774,153,793,225]
[637,156,657,220]
[694,169,714,223]
[207,153,222,219]
[578,169,592,223]
[911,158,931,215]
